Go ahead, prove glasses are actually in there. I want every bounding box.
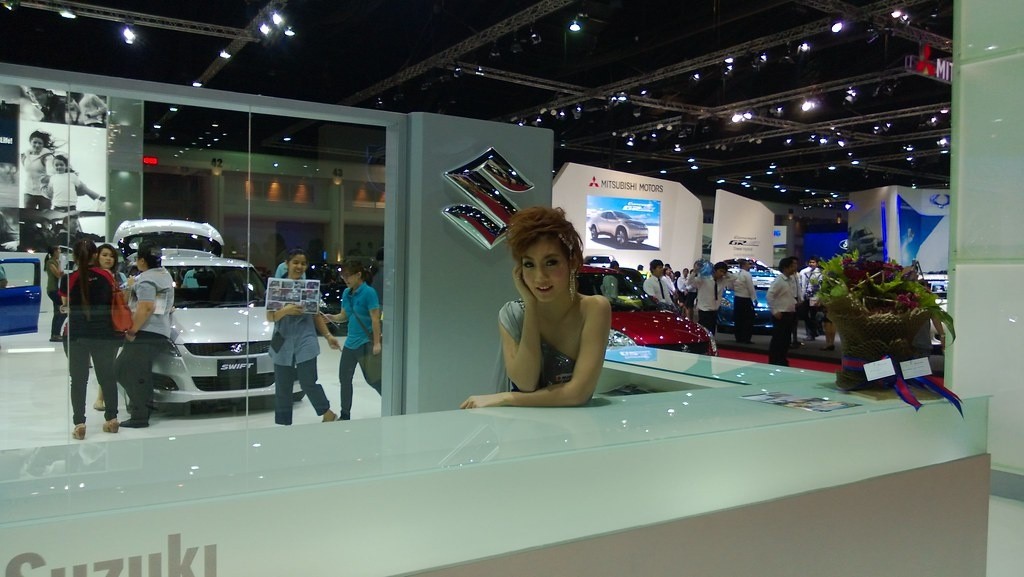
[341,272,358,279]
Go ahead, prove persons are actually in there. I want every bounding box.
[321,259,382,421]
[25,86,107,127]
[603,261,620,298]
[181,266,207,287]
[116,239,174,427]
[766,255,836,367]
[275,262,306,280]
[21,130,106,229]
[643,260,673,305]
[58,238,119,440]
[93,243,127,410]
[44,245,67,342]
[688,261,737,336]
[266,248,342,424]
[459,206,611,409]
[662,264,697,320]
[733,260,757,344]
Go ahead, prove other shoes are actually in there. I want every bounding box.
[94,400,105,410]
[120,418,149,428]
[803,336,815,341]
[49,334,64,341]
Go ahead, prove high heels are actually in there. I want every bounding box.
[104,418,119,433]
[819,345,836,350]
[72,424,86,439]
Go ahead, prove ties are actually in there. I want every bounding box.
[658,278,665,299]
[714,279,719,300]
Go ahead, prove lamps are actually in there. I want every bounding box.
[0,1,955,206]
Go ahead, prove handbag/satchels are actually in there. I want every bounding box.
[367,332,383,347]
[111,280,132,333]
[365,345,380,384]
[809,297,821,306]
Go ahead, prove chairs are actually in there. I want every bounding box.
[211,271,255,302]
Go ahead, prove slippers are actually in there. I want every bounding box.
[322,414,338,422]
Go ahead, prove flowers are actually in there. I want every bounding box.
[812,246,957,348]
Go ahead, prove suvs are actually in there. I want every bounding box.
[588,210,649,247]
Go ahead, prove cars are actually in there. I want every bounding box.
[113,219,305,414]
[703,242,712,249]
[308,264,348,336]
[717,286,776,332]
[577,267,720,355]
[0,259,41,338]
[720,259,786,288]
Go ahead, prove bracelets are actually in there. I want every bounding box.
[325,332,330,338]
